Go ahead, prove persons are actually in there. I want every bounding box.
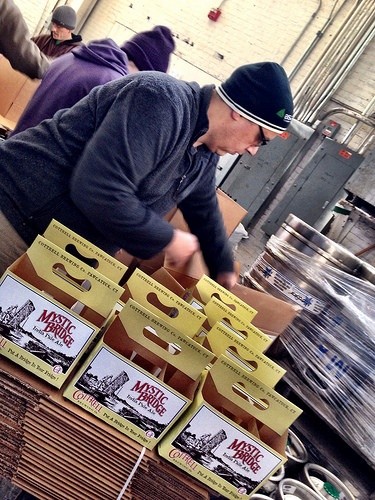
[0,0,175,140]
[0,62,294,289]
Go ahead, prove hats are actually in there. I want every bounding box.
[121,25,175,73]
[215,62,294,134]
[51,6,76,30]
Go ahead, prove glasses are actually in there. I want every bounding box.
[259,126,268,146]
